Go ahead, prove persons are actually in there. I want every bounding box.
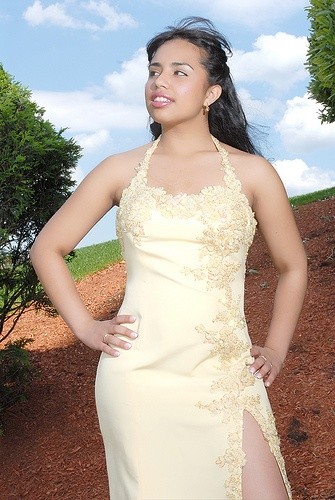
[30,15,307,500]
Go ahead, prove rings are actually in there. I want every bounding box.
[102,333,109,343]
[258,354,267,362]
[265,361,272,366]
